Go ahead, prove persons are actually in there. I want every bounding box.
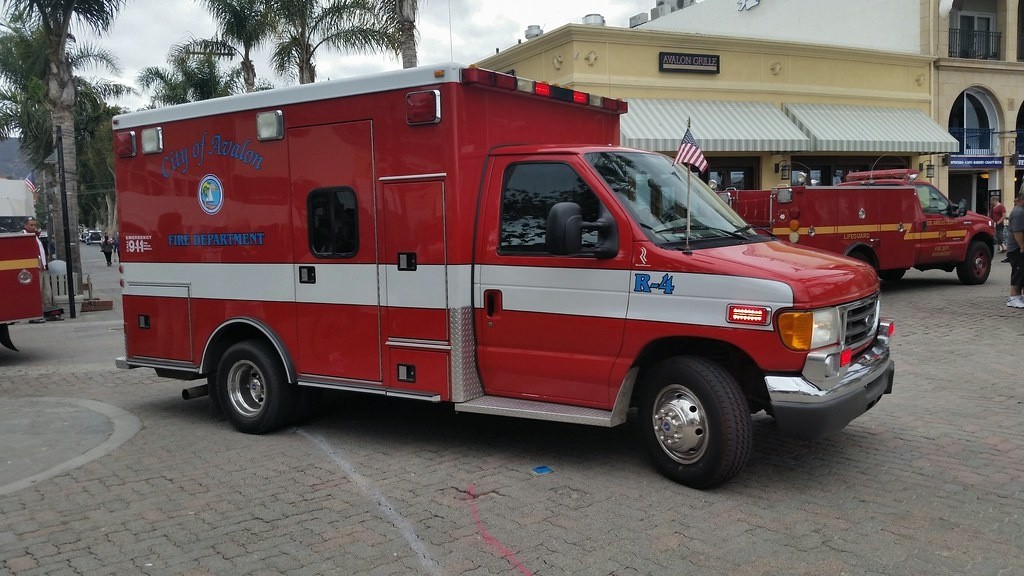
[114,232,120,262]
[19,217,64,323]
[991,197,1007,253]
[1007,191,1024,309]
[101,236,116,266]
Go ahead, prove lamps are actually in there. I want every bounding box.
[919,160,934,177]
[775,160,790,180]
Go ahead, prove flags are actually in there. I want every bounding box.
[672,129,708,173]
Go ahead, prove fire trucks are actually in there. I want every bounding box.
[1,230,46,355]
[708,165,995,286]
[110,62,897,490]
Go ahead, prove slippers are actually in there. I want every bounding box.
[1000,248,1007,253]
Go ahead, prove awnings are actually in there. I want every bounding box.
[784,102,959,154]
[620,98,810,152]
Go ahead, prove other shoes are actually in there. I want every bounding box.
[47,316,64,321]
[29,319,45,323]
[107,262,112,266]
[1001,258,1010,263]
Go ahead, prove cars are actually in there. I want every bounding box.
[78,229,105,245]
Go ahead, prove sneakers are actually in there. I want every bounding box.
[1007,295,1024,308]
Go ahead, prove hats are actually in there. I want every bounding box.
[23,216,35,223]
